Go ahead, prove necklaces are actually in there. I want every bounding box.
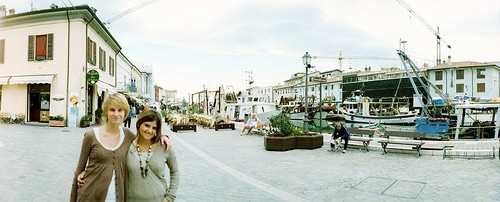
[135,136,154,178]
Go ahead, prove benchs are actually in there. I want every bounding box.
[334,129,374,152]
[0,113,25,124]
[379,131,423,157]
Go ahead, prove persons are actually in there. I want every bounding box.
[240,113,257,136]
[124,100,205,129]
[76,109,181,202]
[327,122,350,153]
[70,93,173,202]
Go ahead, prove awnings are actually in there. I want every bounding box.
[9,74,57,85]
[130,96,148,106]
[0,75,11,86]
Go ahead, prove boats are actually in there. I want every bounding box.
[210,49,500,145]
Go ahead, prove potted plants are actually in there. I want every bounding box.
[95,108,103,125]
[49,116,66,126]
[81,115,91,127]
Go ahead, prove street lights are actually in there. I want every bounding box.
[301,52,312,132]
